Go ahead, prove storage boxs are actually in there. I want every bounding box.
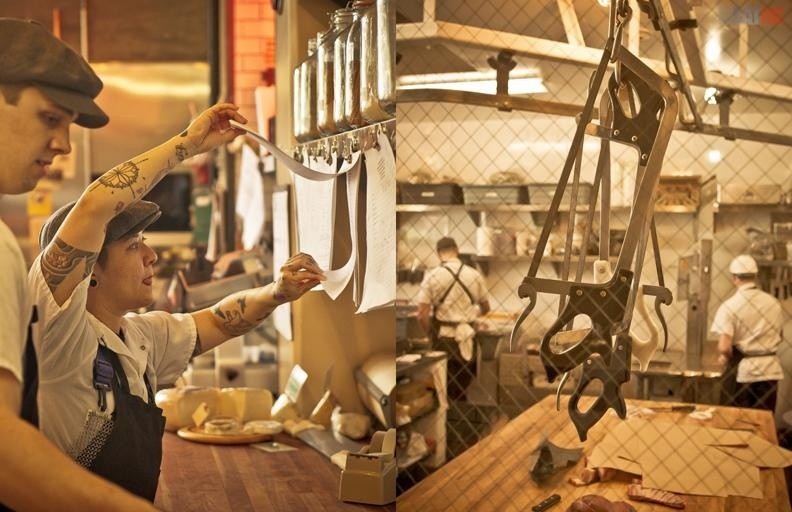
[527,185,592,205]
[401,183,461,204]
[462,185,528,203]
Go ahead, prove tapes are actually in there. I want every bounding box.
[369,428,396,458]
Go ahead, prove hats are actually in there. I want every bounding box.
[438,238,456,251]
[0,18,110,128]
[39,199,162,251]
[730,255,758,274]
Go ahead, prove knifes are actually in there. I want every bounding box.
[647,404,697,414]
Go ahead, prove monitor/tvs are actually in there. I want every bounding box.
[89,171,195,249]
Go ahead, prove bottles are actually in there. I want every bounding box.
[290,0,396,143]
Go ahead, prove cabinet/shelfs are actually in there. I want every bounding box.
[396,204,631,262]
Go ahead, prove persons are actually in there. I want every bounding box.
[417,237,494,401]
[28,101,330,501]
[708,255,782,411]
[0,16,160,512]
